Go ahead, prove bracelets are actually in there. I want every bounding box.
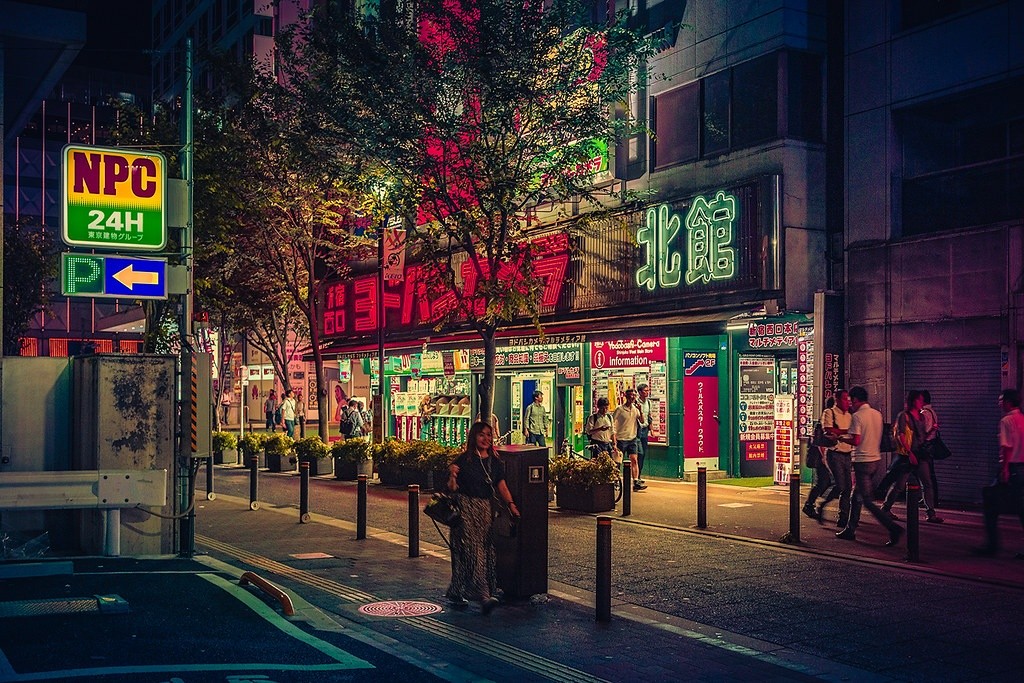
[508,501,514,505]
[906,450,911,455]
[449,476,457,478]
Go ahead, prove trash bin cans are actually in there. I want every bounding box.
[489,445,550,603]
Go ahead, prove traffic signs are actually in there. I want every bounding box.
[61,253,168,300]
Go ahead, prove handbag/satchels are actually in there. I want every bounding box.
[891,412,914,454]
[933,438,951,460]
[920,441,934,458]
[423,488,460,528]
[806,443,819,469]
[813,408,838,448]
[879,422,897,452]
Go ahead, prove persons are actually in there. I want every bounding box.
[815,390,852,527]
[875,389,945,523]
[523,391,549,447]
[586,397,618,460]
[636,383,655,483]
[612,388,648,492]
[802,397,834,518]
[341,400,373,438]
[476,413,499,439]
[334,385,350,421]
[221,388,231,426]
[263,389,307,439]
[970,389,1024,561]
[419,396,435,416]
[836,386,905,547]
[447,422,520,613]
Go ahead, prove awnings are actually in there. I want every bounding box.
[302,305,763,361]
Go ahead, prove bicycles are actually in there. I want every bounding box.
[493,430,535,447]
[547,437,623,511]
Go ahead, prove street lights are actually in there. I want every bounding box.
[366,175,395,442]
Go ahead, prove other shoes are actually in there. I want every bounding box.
[448,595,470,606]
[926,516,943,522]
[633,483,647,492]
[973,545,997,558]
[836,529,855,539]
[881,506,898,519]
[836,518,847,526]
[483,597,499,613]
[802,506,818,519]
[886,527,904,546]
[1015,551,1024,560]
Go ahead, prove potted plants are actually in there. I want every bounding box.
[293,435,334,474]
[371,437,457,489]
[237,431,269,466]
[329,436,374,480]
[548,450,622,513]
[265,430,298,471]
[211,430,239,464]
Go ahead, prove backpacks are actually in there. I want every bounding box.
[339,411,357,435]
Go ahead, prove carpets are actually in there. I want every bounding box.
[706,475,775,488]
